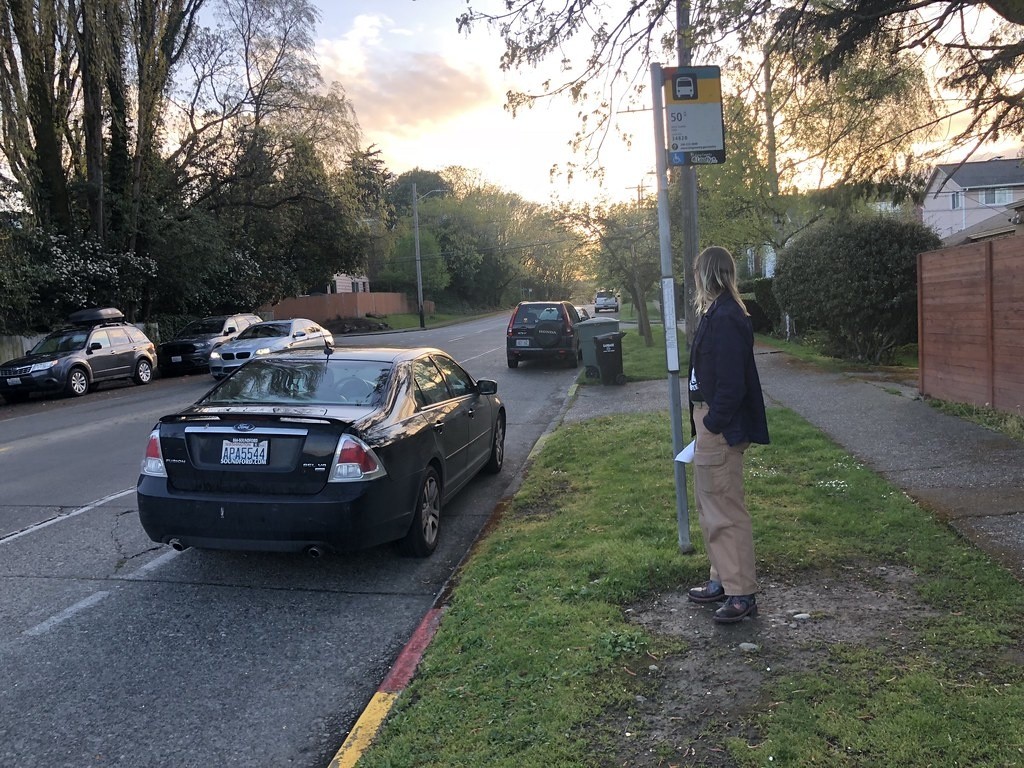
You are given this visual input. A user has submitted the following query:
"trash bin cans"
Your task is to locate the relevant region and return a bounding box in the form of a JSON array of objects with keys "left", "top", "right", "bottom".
[
  {"left": 592, "top": 332, "right": 628, "bottom": 386},
  {"left": 574, "top": 317, "right": 621, "bottom": 380}
]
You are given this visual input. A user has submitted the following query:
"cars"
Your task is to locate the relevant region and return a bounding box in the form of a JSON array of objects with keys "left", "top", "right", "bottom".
[
  {"left": 538, "top": 307, "right": 590, "bottom": 326},
  {"left": 154, "top": 311, "right": 261, "bottom": 373},
  {"left": 208, "top": 318, "right": 335, "bottom": 382},
  {"left": 135, "top": 339, "right": 506, "bottom": 564}
]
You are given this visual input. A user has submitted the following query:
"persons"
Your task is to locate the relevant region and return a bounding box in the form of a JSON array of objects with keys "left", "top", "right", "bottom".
[{"left": 683, "top": 243, "right": 772, "bottom": 624}]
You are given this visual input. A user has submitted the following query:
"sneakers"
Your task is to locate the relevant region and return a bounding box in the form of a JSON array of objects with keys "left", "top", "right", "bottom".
[
  {"left": 687, "top": 583, "right": 727, "bottom": 602},
  {"left": 714, "top": 596, "right": 758, "bottom": 623}
]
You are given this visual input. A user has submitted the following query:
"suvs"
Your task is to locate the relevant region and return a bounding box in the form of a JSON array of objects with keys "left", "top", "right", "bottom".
[
  {"left": 593, "top": 289, "right": 621, "bottom": 312},
  {"left": 0, "top": 306, "right": 158, "bottom": 402},
  {"left": 505, "top": 300, "right": 581, "bottom": 369}
]
